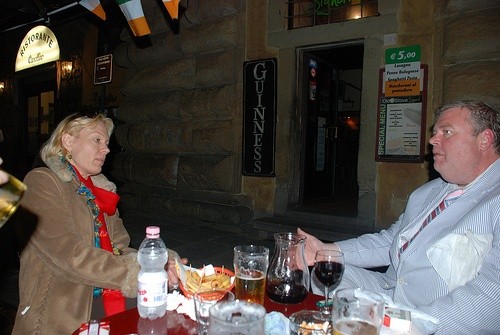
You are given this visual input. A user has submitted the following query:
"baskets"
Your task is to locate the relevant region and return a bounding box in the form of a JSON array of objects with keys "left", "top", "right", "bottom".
[{"left": 180, "top": 266, "right": 236, "bottom": 301}]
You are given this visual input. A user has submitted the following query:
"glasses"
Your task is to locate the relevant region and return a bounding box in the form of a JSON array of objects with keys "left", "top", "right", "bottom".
[{"left": 67, "top": 109, "right": 98, "bottom": 124}]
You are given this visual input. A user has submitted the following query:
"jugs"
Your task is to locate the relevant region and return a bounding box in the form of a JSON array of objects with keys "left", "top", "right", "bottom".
[{"left": 266, "top": 231, "right": 310, "bottom": 304}]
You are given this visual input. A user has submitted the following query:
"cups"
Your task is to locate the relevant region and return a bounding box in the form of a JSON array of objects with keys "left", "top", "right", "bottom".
[
  {"left": 332, "top": 287, "right": 386, "bottom": 335},
  {"left": 289, "top": 310, "right": 332, "bottom": 335},
  {"left": 194, "top": 289, "right": 234, "bottom": 335},
  {"left": 209, "top": 299, "right": 266, "bottom": 335},
  {"left": 232, "top": 244, "right": 269, "bottom": 307},
  {"left": 0, "top": 170, "right": 27, "bottom": 228}
]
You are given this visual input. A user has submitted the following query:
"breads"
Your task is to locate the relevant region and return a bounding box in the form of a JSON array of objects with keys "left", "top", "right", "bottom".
[{"left": 186, "top": 270, "right": 230, "bottom": 298}]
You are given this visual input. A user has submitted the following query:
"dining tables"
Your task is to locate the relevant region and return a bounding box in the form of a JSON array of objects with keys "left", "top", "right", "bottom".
[{"left": 73, "top": 283, "right": 331, "bottom": 335}]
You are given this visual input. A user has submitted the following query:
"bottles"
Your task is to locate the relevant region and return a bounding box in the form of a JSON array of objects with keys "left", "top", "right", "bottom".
[{"left": 136, "top": 226, "right": 168, "bottom": 320}]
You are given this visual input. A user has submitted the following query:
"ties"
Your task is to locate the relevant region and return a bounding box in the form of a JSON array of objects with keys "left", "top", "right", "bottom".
[{"left": 399, "top": 188, "right": 461, "bottom": 254}]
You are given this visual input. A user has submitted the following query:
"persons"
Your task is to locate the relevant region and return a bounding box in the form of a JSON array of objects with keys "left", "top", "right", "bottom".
[
  {"left": 8, "top": 111, "right": 188, "bottom": 335},
  {"left": 284, "top": 100, "right": 500, "bottom": 335}
]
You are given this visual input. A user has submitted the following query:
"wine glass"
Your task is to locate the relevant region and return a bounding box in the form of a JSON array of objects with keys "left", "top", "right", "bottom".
[{"left": 314, "top": 249, "right": 345, "bottom": 321}]
